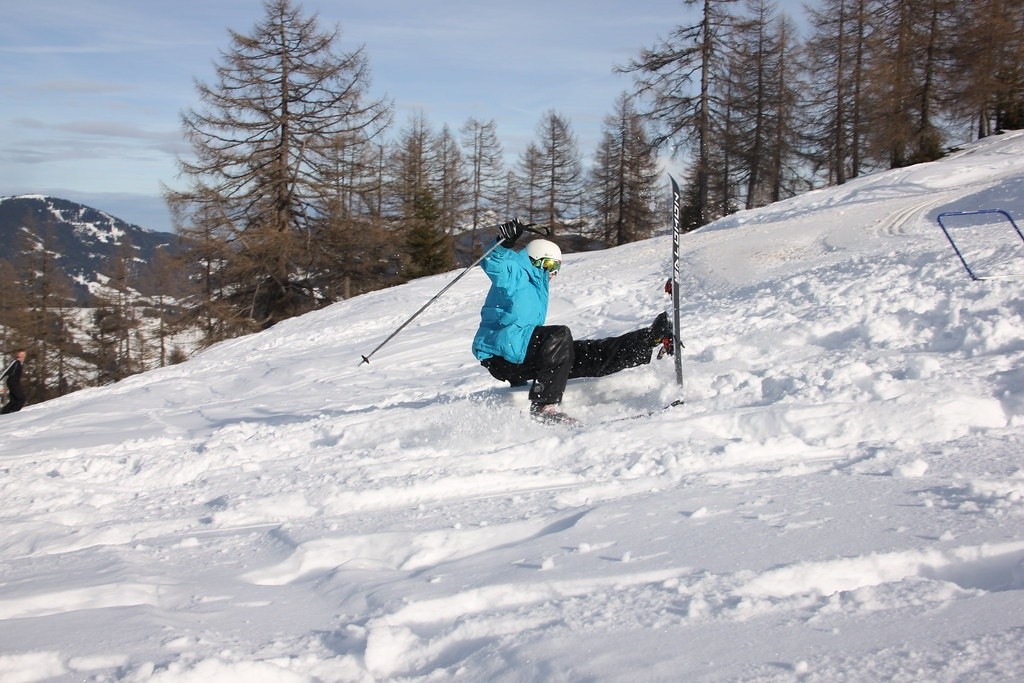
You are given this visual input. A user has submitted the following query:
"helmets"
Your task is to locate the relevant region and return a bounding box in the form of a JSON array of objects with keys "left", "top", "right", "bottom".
[{"left": 525, "top": 239, "right": 562, "bottom": 267}]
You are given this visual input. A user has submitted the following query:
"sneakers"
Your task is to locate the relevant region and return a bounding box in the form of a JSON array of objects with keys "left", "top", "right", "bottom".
[
  {"left": 651, "top": 311, "right": 674, "bottom": 359},
  {"left": 530, "top": 401, "right": 583, "bottom": 430}
]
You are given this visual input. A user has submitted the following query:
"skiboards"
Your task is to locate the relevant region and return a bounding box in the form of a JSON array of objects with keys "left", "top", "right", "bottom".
[
  {"left": 489, "top": 398, "right": 686, "bottom": 456},
  {"left": 666, "top": 171, "right": 685, "bottom": 385}
]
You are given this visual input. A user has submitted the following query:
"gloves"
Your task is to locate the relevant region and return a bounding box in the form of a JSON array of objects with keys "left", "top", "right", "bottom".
[{"left": 496, "top": 218, "right": 523, "bottom": 248}]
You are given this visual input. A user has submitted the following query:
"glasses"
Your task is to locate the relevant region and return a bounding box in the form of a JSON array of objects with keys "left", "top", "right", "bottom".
[{"left": 535, "top": 258, "right": 561, "bottom": 273}]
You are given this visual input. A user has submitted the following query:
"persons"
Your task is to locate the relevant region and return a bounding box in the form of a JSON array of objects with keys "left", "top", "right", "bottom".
[
  {"left": 0, "top": 351, "right": 26, "bottom": 415},
  {"left": 470, "top": 217, "right": 673, "bottom": 427}
]
